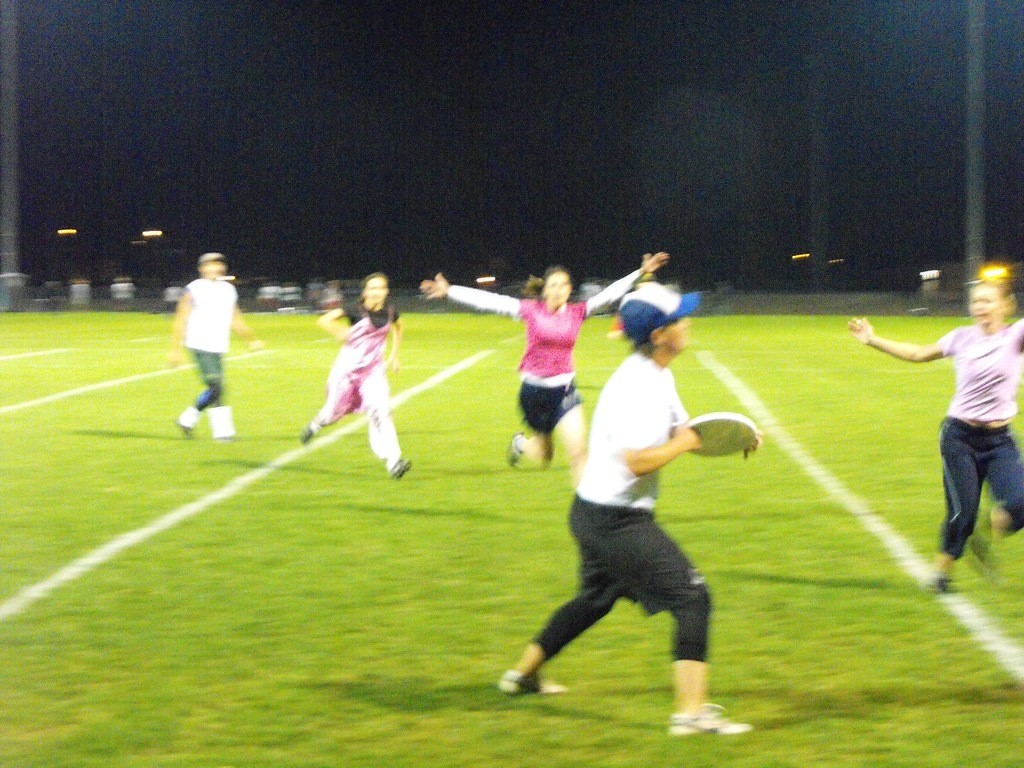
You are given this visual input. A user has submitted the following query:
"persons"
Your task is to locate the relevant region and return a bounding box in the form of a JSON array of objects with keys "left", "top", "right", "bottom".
[
  {"left": 419, "top": 250, "right": 673, "bottom": 468},
  {"left": 298, "top": 271, "right": 412, "bottom": 480},
  {"left": 495, "top": 281, "right": 768, "bottom": 736},
  {"left": 168, "top": 252, "right": 254, "bottom": 442},
  {"left": 847, "top": 263, "right": 1024, "bottom": 593}
]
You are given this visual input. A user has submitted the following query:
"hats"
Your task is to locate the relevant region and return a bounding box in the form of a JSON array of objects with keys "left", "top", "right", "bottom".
[
  {"left": 196, "top": 253, "right": 226, "bottom": 264},
  {"left": 618, "top": 281, "right": 703, "bottom": 344}
]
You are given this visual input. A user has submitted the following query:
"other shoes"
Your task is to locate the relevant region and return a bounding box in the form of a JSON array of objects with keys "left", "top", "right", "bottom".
[
  {"left": 506, "top": 431, "right": 524, "bottom": 467},
  {"left": 301, "top": 425, "right": 313, "bottom": 445},
  {"left": 390, "top": 459, "right": 412, "bottom": 479},
  {"left": 174, "top": 419, "right": 193, "bottom": 439},
  {"left": 923, "top": 572, "right": 950, "bottom": 592},
  {"left": 971, "top": 536, "right": 996, "bottom": 570}
]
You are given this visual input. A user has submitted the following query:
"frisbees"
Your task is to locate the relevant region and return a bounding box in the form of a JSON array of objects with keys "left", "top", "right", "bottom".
[{"left": 684, "top": 411, "right": 761, "bottom": 458}]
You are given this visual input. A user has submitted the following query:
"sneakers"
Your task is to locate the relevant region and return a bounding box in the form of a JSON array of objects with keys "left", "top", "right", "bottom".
[
  {"left": 499, "top": 670, "right": 569, "bottom": 698},
  {"left": 670, "top": 703, "right": 754, "bottom": 738}
]
